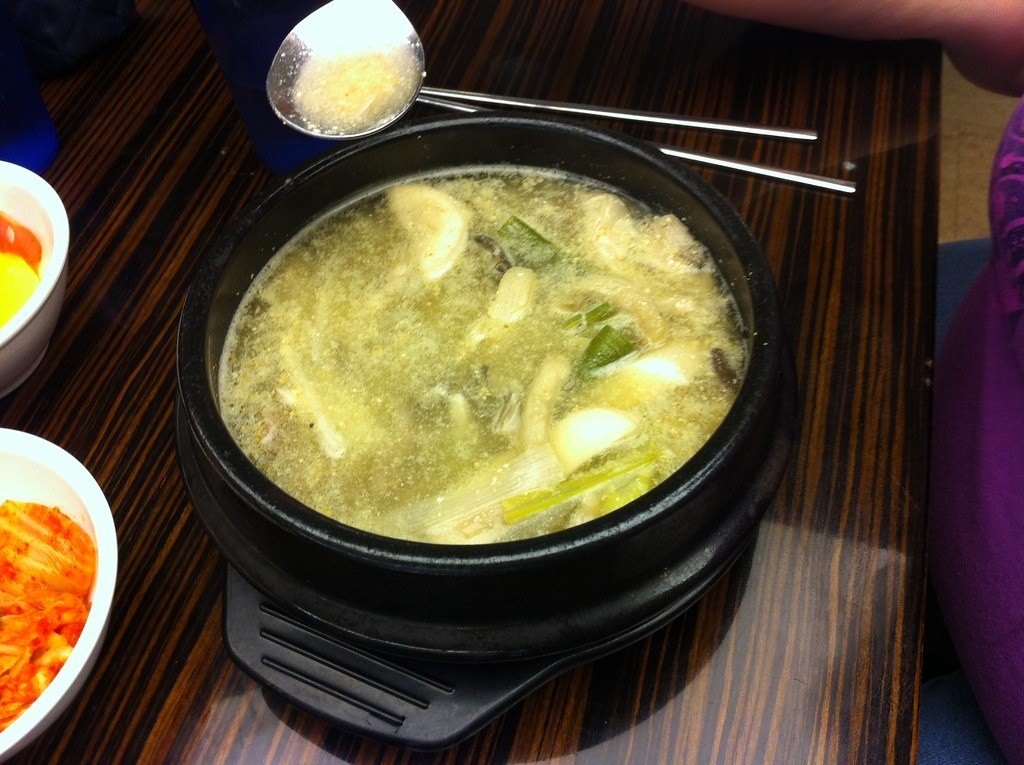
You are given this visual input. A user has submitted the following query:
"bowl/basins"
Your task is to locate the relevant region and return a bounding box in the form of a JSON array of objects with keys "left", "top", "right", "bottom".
[
  {"left": 0, "top": 420, "right": 123, "bottom": 763},
  {"left": 176, "top": 112, "right": 770, "bottom": 589},
  {"left": 0, "top": 154, "right": 71, "bottom": 401}
]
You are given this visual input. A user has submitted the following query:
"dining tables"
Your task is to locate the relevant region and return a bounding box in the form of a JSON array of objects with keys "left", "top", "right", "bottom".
[{"left": 0, "top": 0, "right": 944, "bottom": 765}]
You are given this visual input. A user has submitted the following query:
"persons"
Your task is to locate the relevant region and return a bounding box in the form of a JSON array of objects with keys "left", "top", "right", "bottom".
[{"left": 692, "top": 0, "right": 1024, "bottom": 764}]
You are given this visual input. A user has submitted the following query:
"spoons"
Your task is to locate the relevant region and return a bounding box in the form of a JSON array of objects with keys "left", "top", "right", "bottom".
[{"left": 268, "top": 0, "right": 861, "bottom": 196}]
